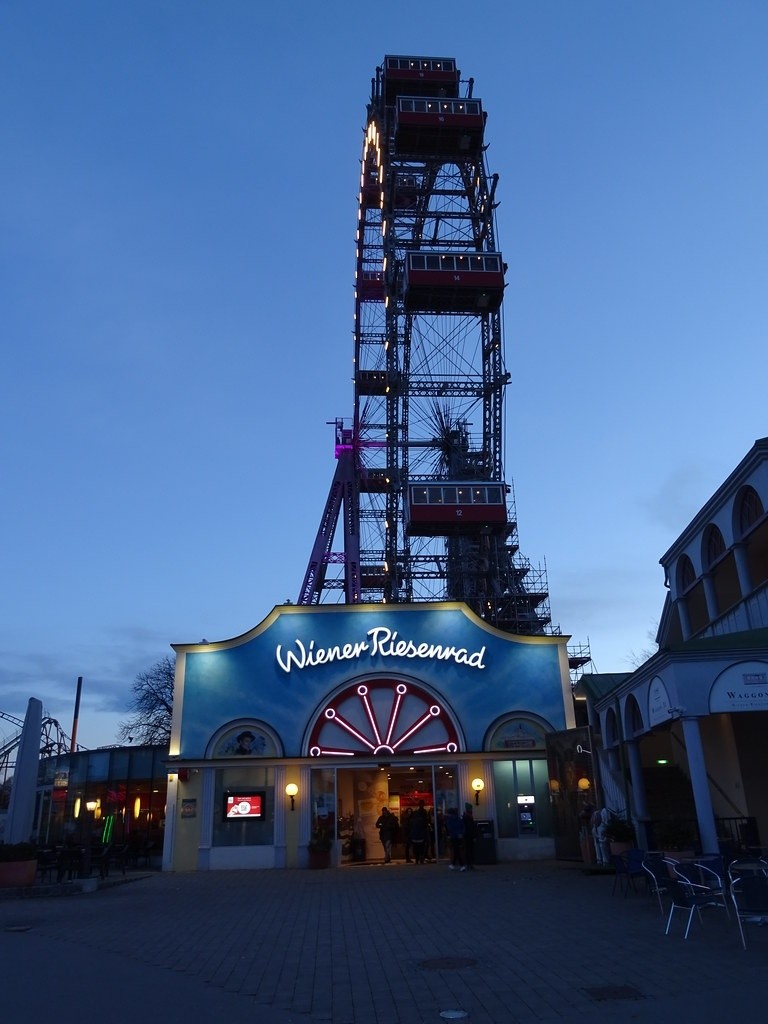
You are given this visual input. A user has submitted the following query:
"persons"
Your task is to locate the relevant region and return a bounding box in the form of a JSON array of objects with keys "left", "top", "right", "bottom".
[
  {"left": 375, "top": 797, "right": 480, "bottom": 872},
  {"left": 236, "top": 730, "right": 257, "bottom": 755}
]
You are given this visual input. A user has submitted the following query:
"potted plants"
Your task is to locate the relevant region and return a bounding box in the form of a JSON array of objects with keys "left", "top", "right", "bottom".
[
  {"left": 0, "top": 840, "right": 46, "bottom": 887},
  {"left": 603, "top": 807, "right": 631, "bottom": 853}
]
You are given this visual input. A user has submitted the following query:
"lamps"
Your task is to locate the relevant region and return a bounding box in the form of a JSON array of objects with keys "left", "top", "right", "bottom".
[
  {"left": 285, "top": 783, "right": 298, "bottom": 811},
  {"left": 577, "top": 776, "right": 592, "bottom": 800},
  {"left": 471, "top": 777, "right": 484, "bottom": 806}
]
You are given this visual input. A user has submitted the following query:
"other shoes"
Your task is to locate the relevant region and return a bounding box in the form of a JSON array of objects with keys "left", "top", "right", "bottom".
[
  {"left": 460, "top": 867, "right": 465, "bottom": 872},
  {"left": 449, "top": 864, "right": 455, "bottom": 870}
]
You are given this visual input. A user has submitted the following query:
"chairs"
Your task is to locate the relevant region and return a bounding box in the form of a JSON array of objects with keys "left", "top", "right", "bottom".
[
  {"left": 641, "top": 856, "right": 702, "bottom": 920},
  {"left": 608, "top": 853, "right": 637, "bottom": 897},
  {"left": 727, "top": 859, "right": 768, "bottom": 951},
  {"left": 666, "top": 859, "right": 739, "bottom": 940},
  {"left": 621, "top": 848, "right": 651, "bottom": 898},
  {"left": 26, "top": 834, "right": 128, "bottom": 880}
]
